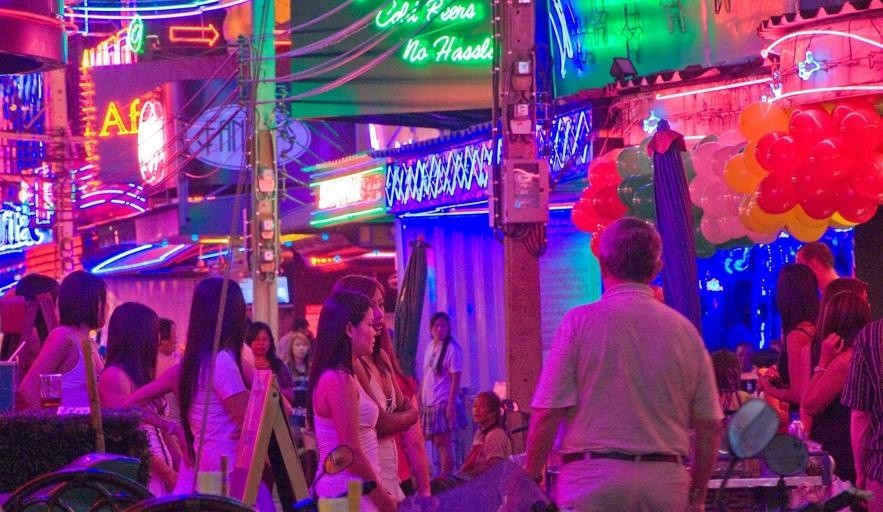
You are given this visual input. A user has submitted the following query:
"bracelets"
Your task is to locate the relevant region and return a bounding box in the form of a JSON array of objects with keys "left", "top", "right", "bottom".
[{"left": 533, "top": 476, "right": 542, "bottom": 485}]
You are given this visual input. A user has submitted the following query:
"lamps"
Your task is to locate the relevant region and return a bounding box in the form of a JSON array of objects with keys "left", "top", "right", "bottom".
[
  {"left": 211, "top": 243, "right": 226, "bottom": 269},
  {"left": 192, "top": 244, "right": 210, "bottom": 273},
  {"left": 610, "top": 57, "right": 638, "bottom": 82}
]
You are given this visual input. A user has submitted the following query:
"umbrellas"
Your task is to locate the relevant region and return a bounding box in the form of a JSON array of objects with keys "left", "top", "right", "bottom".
[{"left": 644, "top": 119, "right": 704, "bottom": 337}]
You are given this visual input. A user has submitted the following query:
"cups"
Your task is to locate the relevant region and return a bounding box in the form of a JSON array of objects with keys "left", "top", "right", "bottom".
[
  {"left": 38, "top": 372, "right": 63, "bottom": 411},
  {"left": 0, "top": 360, "right": 17, "bottom": 410}
]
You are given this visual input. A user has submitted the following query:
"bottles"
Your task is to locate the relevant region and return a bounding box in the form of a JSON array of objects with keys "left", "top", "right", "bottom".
[{"left": 788, "top": 421, "right": 808, "bottom": 441}]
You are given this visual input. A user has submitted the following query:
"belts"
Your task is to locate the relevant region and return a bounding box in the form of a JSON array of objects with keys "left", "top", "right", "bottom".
[{"left": 560, "top": 450, "right": 683, "bottom": 465}]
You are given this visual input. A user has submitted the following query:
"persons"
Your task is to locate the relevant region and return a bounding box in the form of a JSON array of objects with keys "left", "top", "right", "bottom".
[
  {"left": 1, "top": 272, "right": 433, "bottom": 510},
  {"left": 417, "top": 310, "right": 464, "bottom": 473},
  {"left": 710, "top": 241, "right": 883, "bottom": 512},
  {"left": 506, "top": 215, "right": 724, "bottom": 512},
  {"left": 433, "top": 389, "right": 510, "bottom": 490}
]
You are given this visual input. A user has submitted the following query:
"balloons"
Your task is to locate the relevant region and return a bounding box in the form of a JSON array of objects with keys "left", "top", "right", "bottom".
[{"left": 571, "top": 99, "right": 883, "bottom": 255}]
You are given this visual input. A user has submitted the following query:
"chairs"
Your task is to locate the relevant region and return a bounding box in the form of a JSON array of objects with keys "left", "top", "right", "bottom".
[
  {"left": 121, "top": 493, "right": 262, "bottom": 512},
  {"left": 0, "top": 468, "right": 156, "bottom": 511},
  {"left": 711, "top": 409, "right": 750, "bottom": 479}
]
